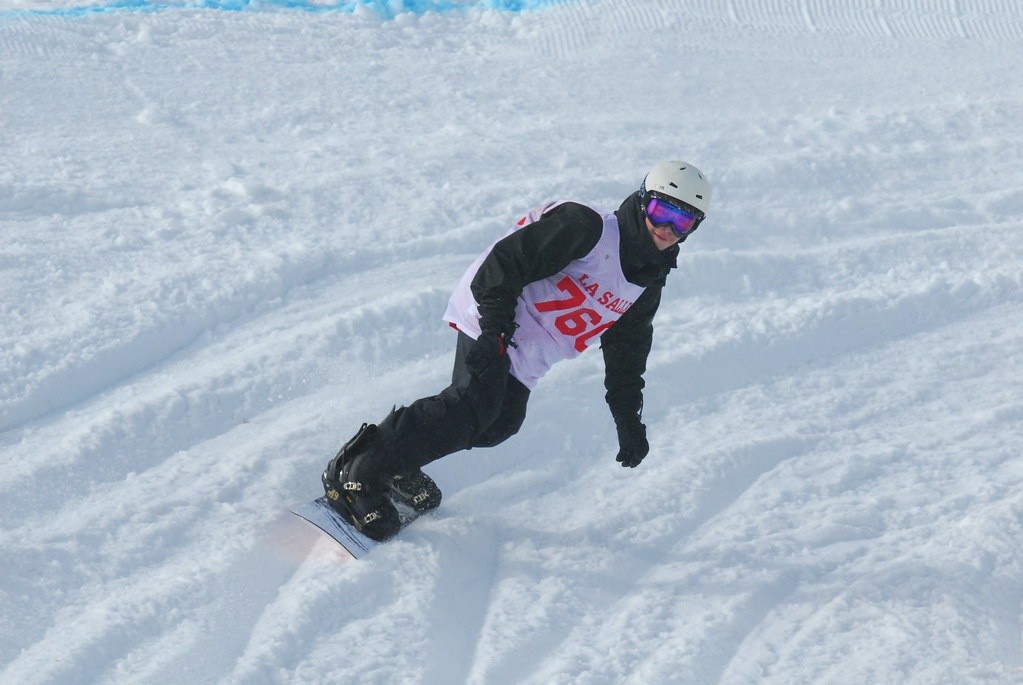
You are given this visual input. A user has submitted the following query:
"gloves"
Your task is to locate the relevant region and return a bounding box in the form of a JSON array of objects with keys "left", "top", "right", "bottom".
[
  {"left": 613, "top": 407, "right": 650, "bottom": 468},
  {"left": 464, "top": 316, "right": 520, "bottom": 367}
]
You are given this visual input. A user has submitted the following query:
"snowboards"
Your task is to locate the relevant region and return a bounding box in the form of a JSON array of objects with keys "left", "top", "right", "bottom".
[{"left": 287, "top": 460, "right": 457, "bottom": 563}]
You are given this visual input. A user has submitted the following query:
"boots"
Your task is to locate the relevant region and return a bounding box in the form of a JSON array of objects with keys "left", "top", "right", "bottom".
[
  {"left": 321, "top": 404, "right": 409, "bottom": 540},
  {"left": 386, "top": 466, "right": 442, "bottom": 514}
]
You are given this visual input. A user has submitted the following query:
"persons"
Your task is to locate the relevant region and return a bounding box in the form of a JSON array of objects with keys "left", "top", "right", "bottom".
[{"left": 322, "top": 159, "right": 712, "bottom": 542}]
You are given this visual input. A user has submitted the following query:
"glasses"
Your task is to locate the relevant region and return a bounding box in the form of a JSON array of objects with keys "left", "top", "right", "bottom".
[{"left": 644, "top": 196, "right": 697, "bottom": 239}]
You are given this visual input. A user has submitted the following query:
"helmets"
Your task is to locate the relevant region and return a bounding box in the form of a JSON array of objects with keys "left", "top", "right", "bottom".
[{"left": 641, "top": 160, "right": 711, "bottom": 230}]
